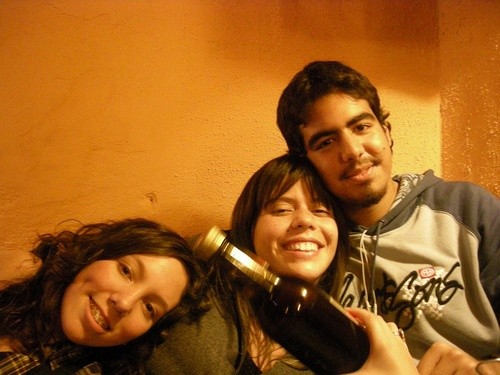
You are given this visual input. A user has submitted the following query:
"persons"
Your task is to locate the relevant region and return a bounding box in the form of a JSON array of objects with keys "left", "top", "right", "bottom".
[
  {"left": 276, "top": 60, "right": 500, "bottom": 375},
  {"left": 148, "top": 153, "right": 418, "bottom": 375},
  {"left": 1, "top": 218, "right": 213, "bottom": 375}
]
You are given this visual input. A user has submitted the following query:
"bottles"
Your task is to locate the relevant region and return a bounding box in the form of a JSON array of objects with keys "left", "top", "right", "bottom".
[{"left": 192, "top": 226, "right": 372, "bottom": 375}]
